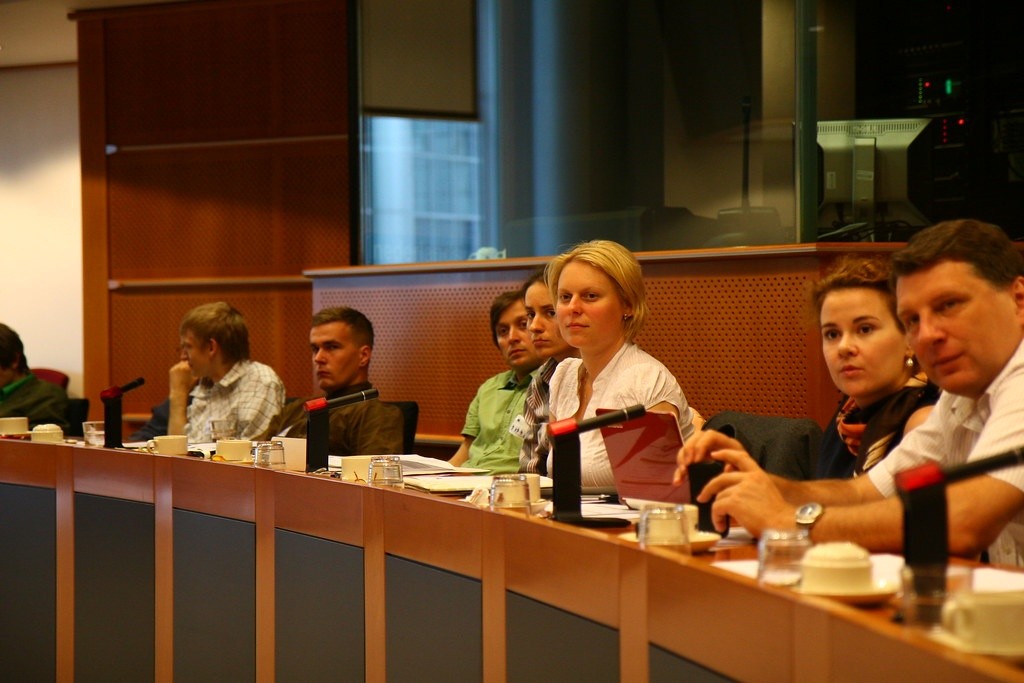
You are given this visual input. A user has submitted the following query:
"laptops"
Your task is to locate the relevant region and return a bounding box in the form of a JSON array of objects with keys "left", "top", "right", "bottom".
[{"left": 596, "top": 409, "right": 692, "bottom": 507}]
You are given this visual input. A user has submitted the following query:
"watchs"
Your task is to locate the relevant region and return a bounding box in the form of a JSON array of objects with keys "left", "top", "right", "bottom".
[{"left": 795, "top": 502, "right": 825, "bottom": 539}]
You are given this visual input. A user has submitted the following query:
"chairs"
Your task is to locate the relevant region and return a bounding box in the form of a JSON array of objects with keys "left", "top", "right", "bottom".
[
  {"left": 63, "top": 397, "right": 91, "bottom": 439},
  {"left": 383, "top": 401, "right": 419, "bottom": 454},
  {"left": 698, "top": 411, "right": 826, "bottom": 482}
]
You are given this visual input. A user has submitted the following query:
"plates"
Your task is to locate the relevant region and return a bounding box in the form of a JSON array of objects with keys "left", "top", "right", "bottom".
[
  {"left": 3, "top": 431, "right": 34, "bottom": 436},
  {"left": 931, "top": 627, "right": 1024, "bottom": 663},
  {"left": 618, "top": 532, "right": 720, "bottom": 550},
  {"left": 792, "top": 581, "right": 900, "bottom": 604},
  {"left": 529, "top": 500, "right": 553, "bottom": 513}
]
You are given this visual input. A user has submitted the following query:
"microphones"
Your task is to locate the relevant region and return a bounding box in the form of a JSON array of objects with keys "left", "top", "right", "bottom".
[
  {"left": 941, "top": 445, "right": 1024, "bottom": 482},
  {"left": 575, "top": 404, "right": 646, "bottom": 432},
  {"left": 120, "top": 377, "right": 144, "bottom": 393},
  {"left": 328, "top": 388, "right": 379, "bottom": 410}
]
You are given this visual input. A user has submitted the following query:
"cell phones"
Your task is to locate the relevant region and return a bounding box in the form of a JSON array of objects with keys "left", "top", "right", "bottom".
[{"left": 688, "top": 461, "right": 729, "bottom": 538}]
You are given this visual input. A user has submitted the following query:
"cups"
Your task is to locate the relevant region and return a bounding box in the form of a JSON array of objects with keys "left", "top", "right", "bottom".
[
  {"left": 146, "top": 435, "right": 188, "bottom": 455},
  {"left": 799, "top": 539, "right": 874, "bottom": 591},
  {"left": 253, "top": 442, "right": 285, "bottom": 470},
  {"left": 81, "top": 421, "right": 106, "bottom": 448},
  {"left": 215, "top": 440, "right": 252, "bottom": 462},
  {"left": 670, "top": 505, "right": 700, "bottom": 534},
  {"left": 942, "top": 590, "right": 1024, "bottom": 656},
  {"left": 523, "top": 474, "right": 541, "bottom": 502},
  {"left": 367, "top": 456, "right": 406, "bottom": 490},
  {"left": 637, "top": 504, "right": 692, "bottom": 558},
  {"left": 0, "top": 417, "right": 29, "bottom": 435},
  {"left": 490, "top": 474, "right": 532, "bottom": 516},
  {"left": 31, "top": 424, "right": 64, "bottom": 444},
  {"left": 756, "top": 529, "right": 813, "bottom": 588}
]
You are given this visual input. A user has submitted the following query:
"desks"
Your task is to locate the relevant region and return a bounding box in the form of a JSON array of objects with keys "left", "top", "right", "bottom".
[{"left": 1, "top": 439, "right": 1024, "bottom": 683}]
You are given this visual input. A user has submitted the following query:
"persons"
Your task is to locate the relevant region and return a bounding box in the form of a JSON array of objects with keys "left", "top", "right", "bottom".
[
  {"left": 180, "top": 302, "right": 286, "bottom": 444},
  {"left": 250, "top": 304, "right": 405, "bottom": 474},
  {"left": 0, "top": 321, "right": 80, "bottom": 433},
  {"left": 126, "top": 338, "right": 202, "bottom": 443},
  {"left": 544, "top": 238, "right": 696, "bottom": 496},
  {"left": 447, "top": 291, "right": 551, "bottom": 475},
  {"left": 810, "top": 254, "right": 941, "bottom": 479},
  {"left": 519, "top": 273, "right": 582, "bottom": 475},
  {"left": 673, "top": 219, "right": 1024, "bottom": 565}
]
